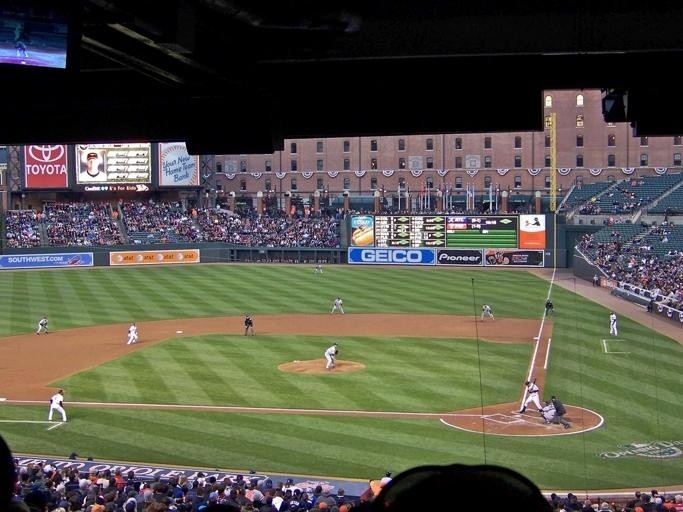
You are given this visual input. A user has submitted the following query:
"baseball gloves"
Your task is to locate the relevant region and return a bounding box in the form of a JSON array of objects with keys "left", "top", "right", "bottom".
[{"left": 334, "top": 350, "right": 337, "bottom": 354}]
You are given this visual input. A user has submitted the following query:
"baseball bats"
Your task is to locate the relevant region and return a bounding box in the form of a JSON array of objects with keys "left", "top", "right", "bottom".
[{"left": 531, "top": 378, "right": 536, "bottom": 390}]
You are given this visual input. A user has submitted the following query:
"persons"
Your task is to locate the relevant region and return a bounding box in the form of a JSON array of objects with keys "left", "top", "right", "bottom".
[
  {"left": 14, "top": 40, "right": 28, "bottom": 58},
  {"left": 79, "top": 152, "right": 107, "bottom": 181}
]
